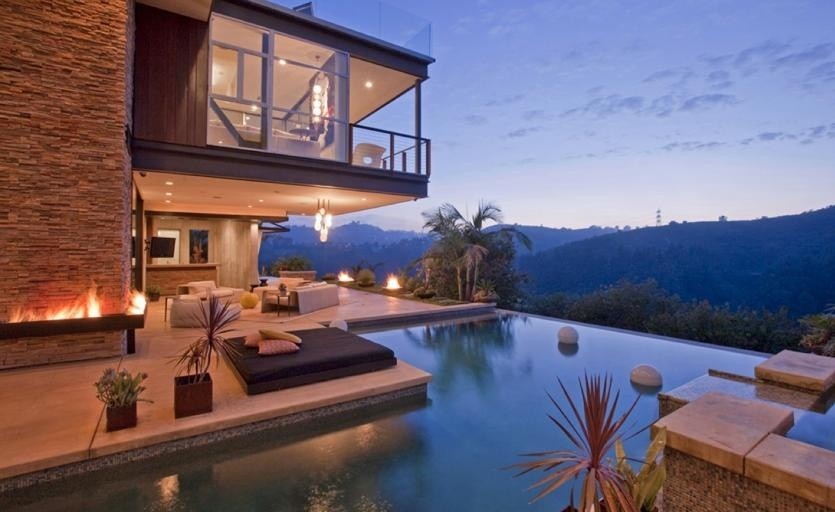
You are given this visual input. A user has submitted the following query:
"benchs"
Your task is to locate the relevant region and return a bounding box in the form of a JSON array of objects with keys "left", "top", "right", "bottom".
[{"left": 163, "top": 278, "right": 341, "bottom": 329}]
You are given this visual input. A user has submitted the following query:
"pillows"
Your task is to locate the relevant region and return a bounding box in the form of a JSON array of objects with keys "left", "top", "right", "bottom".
[{"left": 245, "top": 327, "right": 302, "bottom": 355}]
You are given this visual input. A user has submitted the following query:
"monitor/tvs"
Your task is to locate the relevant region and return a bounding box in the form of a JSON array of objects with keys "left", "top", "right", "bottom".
[{"left": 150, "top": 237, "right": 176, "bottom": 258}]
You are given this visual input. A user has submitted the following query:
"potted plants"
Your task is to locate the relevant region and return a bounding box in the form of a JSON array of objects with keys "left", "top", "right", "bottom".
[{"left": 94, "top": 286, "right": 247, "bottom": 433}]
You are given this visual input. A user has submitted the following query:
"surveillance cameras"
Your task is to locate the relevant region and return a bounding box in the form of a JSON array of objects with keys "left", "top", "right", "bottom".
[{"left": 139, "top": 171, "right": 147, "bottom": 177}]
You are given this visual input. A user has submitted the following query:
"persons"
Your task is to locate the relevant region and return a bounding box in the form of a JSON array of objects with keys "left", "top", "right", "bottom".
[{"left": 192, "top": 239, "right": 204, "bottom": 263}]
